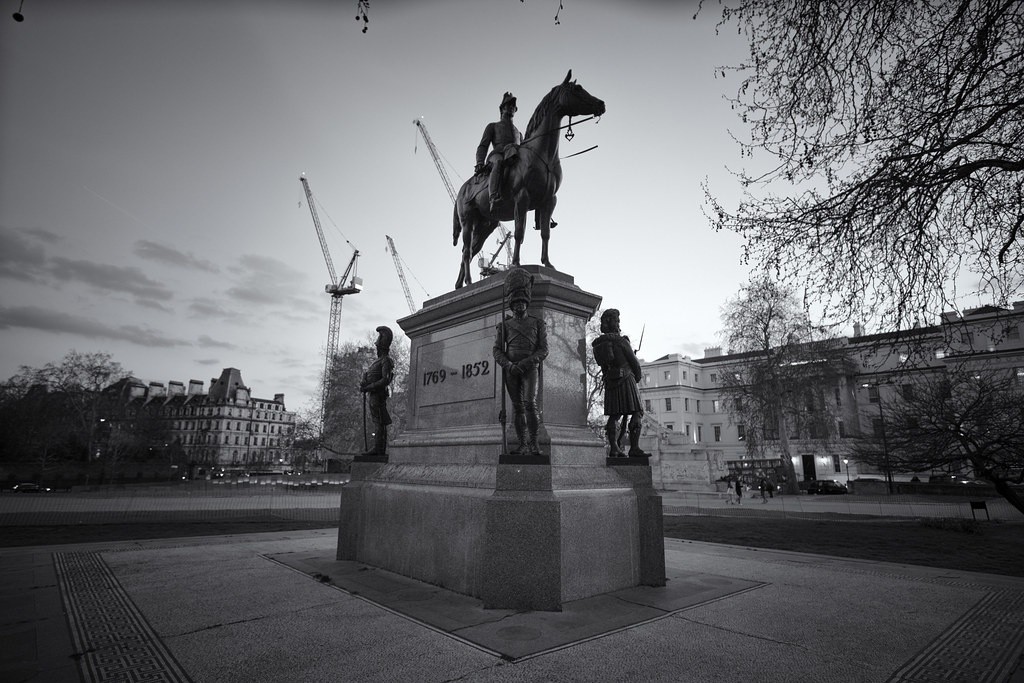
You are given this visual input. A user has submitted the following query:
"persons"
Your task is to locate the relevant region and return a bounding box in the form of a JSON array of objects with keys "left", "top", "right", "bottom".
[
  {"left": 725, "top": 476, "right": 775, "bottom": 506},
  {"left": 359, "top": 327, "right": 394, "bottom": 456},
  {"left": 592, "top": 309, "right": 653, "bottom": 458},
  {"left": 475, "top": 93, "right": 558, "bottom": 230},
  {"left": 491, "top": 268, "right": 549, "bottom": 455}
]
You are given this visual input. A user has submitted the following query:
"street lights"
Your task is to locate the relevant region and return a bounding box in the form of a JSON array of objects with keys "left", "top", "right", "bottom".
[
  {"left": 842, "top": 457, "right": 850, "bottom": 481},
  {"left": 99, "top": 417, "right": 112, "bottom": 485},
  {"left": 244, "top": 401, "right": 257, "bottom": 475},
  {"left": 860, "top": 383, "right": 894, "bottom": 495}
]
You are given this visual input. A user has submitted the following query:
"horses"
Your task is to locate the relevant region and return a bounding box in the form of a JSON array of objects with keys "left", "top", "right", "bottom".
[{"left": 452, "top": 68, "right": 606, "bottom": 290}]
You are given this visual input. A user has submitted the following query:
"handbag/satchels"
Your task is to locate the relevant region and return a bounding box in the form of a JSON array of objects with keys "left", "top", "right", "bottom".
[{"left": 727, "top": 486, "right": 733, "bottom": 495}]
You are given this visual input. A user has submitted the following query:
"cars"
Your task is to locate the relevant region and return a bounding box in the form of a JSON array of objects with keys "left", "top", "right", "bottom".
[
  {"left": 929, "top": 474, "right": 975, "bottom": 486},
  {"left": 12, "top": 483, "right": 52, "bottom": 494},
  {"left": 807, "top": 479, "right": 848, "bottom": 496}
]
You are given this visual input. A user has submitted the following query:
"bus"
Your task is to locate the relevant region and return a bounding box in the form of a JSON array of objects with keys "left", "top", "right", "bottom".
[{"left": 726, "top": 458, "right": 786, "bottom": 492}]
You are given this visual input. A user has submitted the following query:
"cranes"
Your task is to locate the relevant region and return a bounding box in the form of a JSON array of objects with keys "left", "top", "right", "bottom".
[
  {"left": 384, "top": 236, "right": 427, "bottom": 315},
  {"left": 413, "top": 116, "right": 514, "bottom": 281},
  {"left": 297, "top": 174, "right": 361, "bottom": 436}
]
